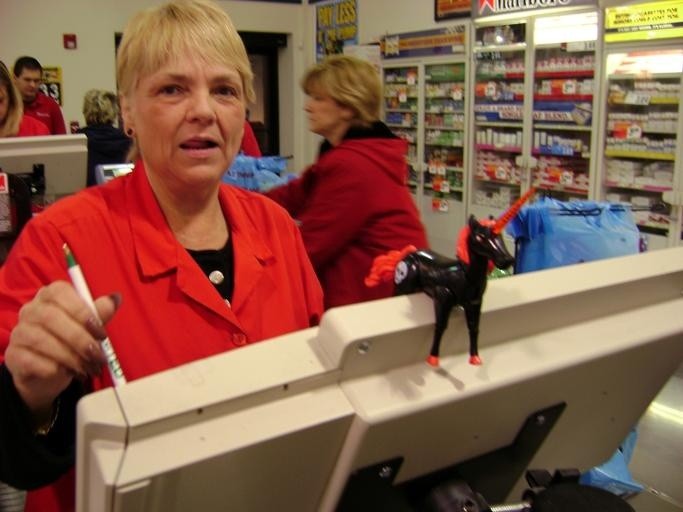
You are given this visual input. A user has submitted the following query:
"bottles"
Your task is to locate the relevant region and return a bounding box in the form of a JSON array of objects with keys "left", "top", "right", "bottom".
[
  {"left": 606, "top": 137, "right": 676, "bottom": 152},
  {"left": 474, "top": 128, "right": 523, "bottom": 149},
  {"left": 531, "top": 130, "right": 584, "bottom": 153},
  {"left": 608, "top": 111, "right": 677, "bottom": 130},
  {"left": 633, "top": 81, "right": 680, "bottom": 97},
  {"left": 28, "top": 162, "right": 46, "bottom": 208}
]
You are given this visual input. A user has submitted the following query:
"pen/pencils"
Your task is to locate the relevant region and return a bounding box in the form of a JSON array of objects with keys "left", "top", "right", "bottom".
[{"left": 62, "top": 242, "right": 128, "bottom": 388}]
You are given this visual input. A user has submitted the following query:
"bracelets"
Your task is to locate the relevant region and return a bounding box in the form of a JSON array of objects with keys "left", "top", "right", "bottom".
[{"left": 35, "top": 401, "right": 60, "bottom": 436}]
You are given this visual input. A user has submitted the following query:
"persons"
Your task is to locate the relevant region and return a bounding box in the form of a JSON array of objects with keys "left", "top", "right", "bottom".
[
  {"left": 13, "top": 56, "right": 66, "bottom": 136},
  {"left": 0, "top": 0, "right": 325, "bottom": 512},
  {"left": 75, "top": 88, "right": 132, "bottom": 188},
  {"left": 260, "top": 54, "right": 429, "bottom": 311},
  {"left": 0, "top": 61, "right": 50, "bottom": 137},
  {"left": 240, "top": 119, "right": 262, "bottom": 158}
]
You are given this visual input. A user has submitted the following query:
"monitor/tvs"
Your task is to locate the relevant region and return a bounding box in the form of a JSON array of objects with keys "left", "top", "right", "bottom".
[
  {"left": 63, "top": 249, "right": 682, "bottom": 512},
  {"left": 0, "top": 134, "right": 94, "bottom": 247}
]
